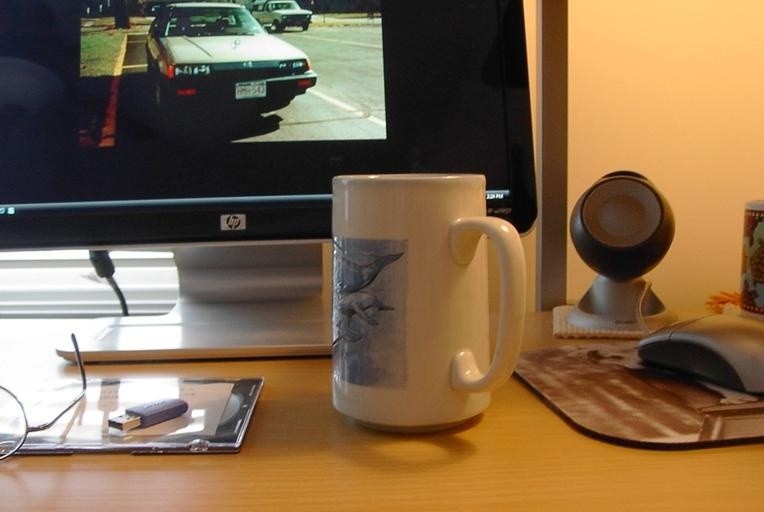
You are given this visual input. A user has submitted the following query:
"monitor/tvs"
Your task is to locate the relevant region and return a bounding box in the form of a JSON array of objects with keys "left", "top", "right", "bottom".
[{"left": 0, "top": 0, "right": 539, "bottom": 363}]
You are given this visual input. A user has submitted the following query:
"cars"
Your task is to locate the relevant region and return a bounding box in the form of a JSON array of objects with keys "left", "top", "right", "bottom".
[{"left": 145, "top": 0, "right": 317, "bottom": 117}]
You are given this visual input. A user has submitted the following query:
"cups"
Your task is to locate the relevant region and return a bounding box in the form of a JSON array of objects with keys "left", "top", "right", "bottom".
[{"left": 333, "top": 175, "right": 526, "bottom": 432}]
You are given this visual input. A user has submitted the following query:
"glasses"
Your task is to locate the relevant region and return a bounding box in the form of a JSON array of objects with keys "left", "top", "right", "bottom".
[{"left": 0, "top": 333, "right": 86, "bottom": 461}]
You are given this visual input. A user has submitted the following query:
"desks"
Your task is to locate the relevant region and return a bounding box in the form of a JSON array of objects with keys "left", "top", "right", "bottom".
[{"left": 0, "top": 297, "right": 761, "bottom": 511}]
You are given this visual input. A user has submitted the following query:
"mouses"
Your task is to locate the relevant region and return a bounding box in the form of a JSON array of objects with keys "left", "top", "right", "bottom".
[{"left": 634, "top": 313, "right": 764, "bottom": 397}]
